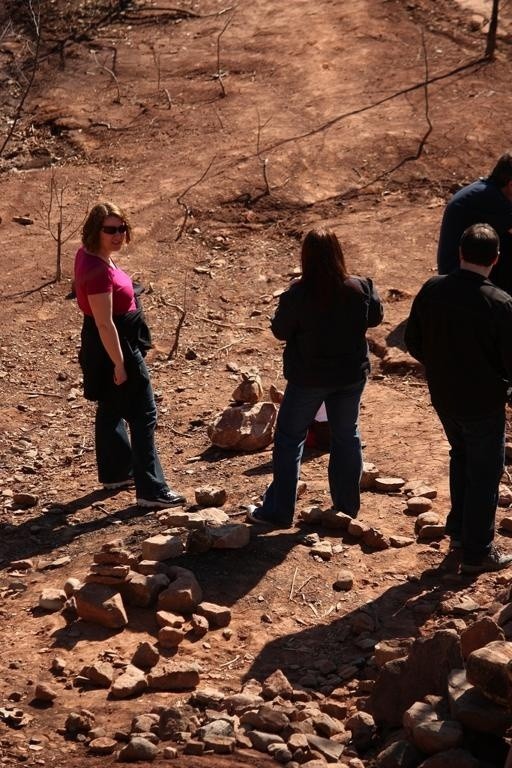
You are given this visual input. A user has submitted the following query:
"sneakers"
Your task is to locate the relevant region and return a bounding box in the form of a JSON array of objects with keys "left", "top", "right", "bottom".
[
  {"left": 100, "top": 469, "right": 138, "bottom": 491},
  {"left": 134, "top": 486, "right": 187, "bottom": 509},
  {"left": 450, "top": 529, "right": 512, "bottom": 574},
  {"left": 246, "top": 506, "right": 293, "bottom": 529}
]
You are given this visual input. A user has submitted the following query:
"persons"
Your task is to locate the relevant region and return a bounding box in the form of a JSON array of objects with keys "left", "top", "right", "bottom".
[
  {"left": 247, "top": 228, "right": 385, "bottom": 531},
  {"left": 70, "top": 202, "right": 187, "bottom": 512},
  {"left": 403, "top": 224, "right": 511, "bottom": 577},
  {"left": 433, "top": 153, "right": 510, "bottom": 295}
]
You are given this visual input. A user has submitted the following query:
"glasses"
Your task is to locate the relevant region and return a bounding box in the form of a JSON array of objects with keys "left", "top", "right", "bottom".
[{"left": 96, "top": 224, "right": 127, "bottom": 235}]
